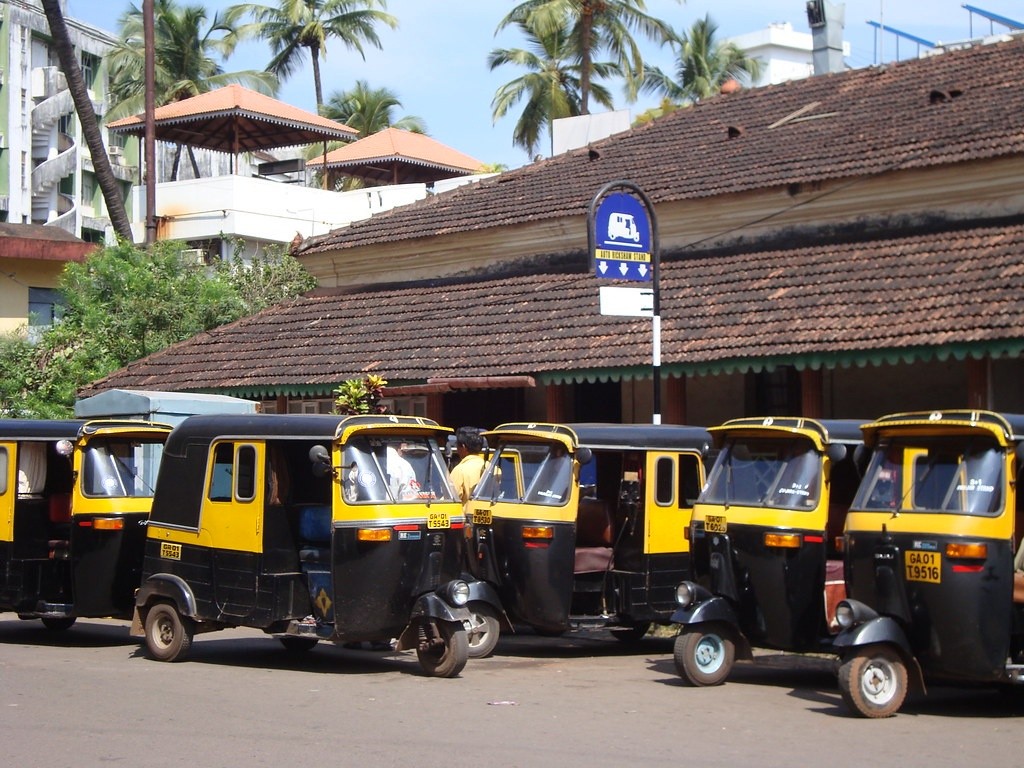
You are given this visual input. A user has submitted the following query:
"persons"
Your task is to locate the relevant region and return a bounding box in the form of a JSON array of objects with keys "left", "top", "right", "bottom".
[
  {"left": 447, "top": 428, "right": 502, "bottom": 554},
  {"left": 386, "top": 443, "right": 417, "bottom": 501}
]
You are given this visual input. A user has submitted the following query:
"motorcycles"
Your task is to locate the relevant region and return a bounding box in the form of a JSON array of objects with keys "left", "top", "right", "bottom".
[
  {"left": 133, "top": 411, "right": 472, "bottom": 680},
  {"left": 0, "top": 418, "right": 175, "bottom": 633},
  {"left": 444, "top": 420, "right": 719, "bottom": 659},
  {"left": 830, "top": 407, "right": 1024, "bottom": 720},
  {"left": 670, "top": 417, "right": 967, "bottom": 690},
  {"left": 401, "top": 447, "right": 525, "bottom": 503}
]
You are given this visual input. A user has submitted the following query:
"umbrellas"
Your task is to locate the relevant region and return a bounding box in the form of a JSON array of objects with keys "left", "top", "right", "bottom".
[
  {"left": 106, "top": 84, "right": 357, "bottom": 177},
  {"left": 304, "top": 127, "right": 487, "bottom": 185}
]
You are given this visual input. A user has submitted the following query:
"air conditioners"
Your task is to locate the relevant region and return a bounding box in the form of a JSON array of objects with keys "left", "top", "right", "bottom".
[
  {"left": 181, "top": 249, "right": 210, "bottom": 266},
  {"left": 108, "top": 146, "right": 123, "bottom": 155}
]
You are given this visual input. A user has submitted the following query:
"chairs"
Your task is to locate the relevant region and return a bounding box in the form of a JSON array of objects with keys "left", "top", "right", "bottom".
[
  {"left": 826, "top": 503, "right": 849, "bottom": 581},
  {"left": 1013, "top": 510, "right": 1024, "bottom": 603},
  {"left": 43, "top": 492, "right": 73, "bottom": 548},
  {"left": 298, "top": 505, "right": 331, "bottom": 564},
  {"left": 575, "top": 497, "right": 615, "bottom": 574}
]
